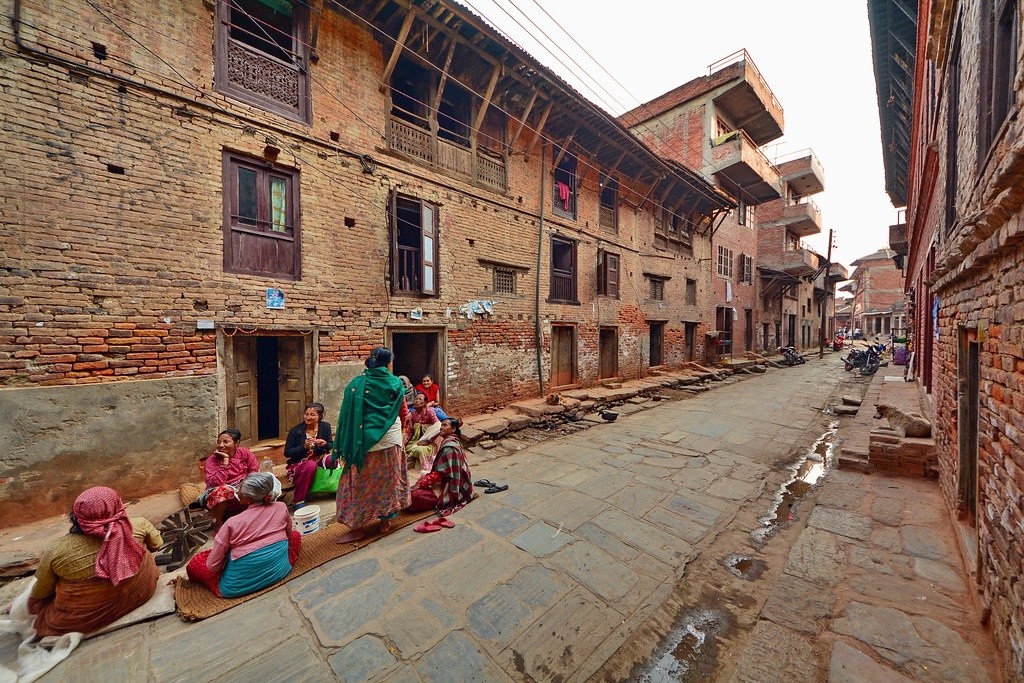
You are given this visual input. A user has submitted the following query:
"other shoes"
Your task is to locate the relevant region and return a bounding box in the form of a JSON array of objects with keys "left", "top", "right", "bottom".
[{"left": 294, "top": 503, "right": 305, "bottom": 511}]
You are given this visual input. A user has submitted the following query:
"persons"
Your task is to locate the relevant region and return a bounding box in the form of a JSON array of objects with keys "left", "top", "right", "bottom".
[
  {"left": 283, "top": 402, "right": 338, "bottom": 510},
  {"left": 401, "top": 417, "right": 478, "bottom": 517},
  {"left": 203, "top": 428, "right": 260, "bottom": 537},
  {"left": 27, "top": 486, "right": 164, "bottom": 638},
  {"left": 414, "top": 374, "right": 449, "bottom": 420},
  {"left": 331, "top": 346, "right": 412, "bottom": 544},
  {"left": 185, "top": 472, "right": 301, "bottom": 599},
  {"left": 398, "top": 392, "right": 444, "bottom": 474},
  {"left": 397, "top": 375, "right": 417, "bottom": 414}
]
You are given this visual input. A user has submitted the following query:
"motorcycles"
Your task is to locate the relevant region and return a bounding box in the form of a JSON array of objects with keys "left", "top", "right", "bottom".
[
  {"left": 777, "top": 338, "right": 806, "bottom": 366},
  {"left": 840, "top": 336, "right": 887, "bottom": 376}
]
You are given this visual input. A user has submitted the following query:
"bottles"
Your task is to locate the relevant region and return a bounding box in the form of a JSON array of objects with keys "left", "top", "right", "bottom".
[{"left": 261, "top": 456, "right": 273, "bottom": 473}]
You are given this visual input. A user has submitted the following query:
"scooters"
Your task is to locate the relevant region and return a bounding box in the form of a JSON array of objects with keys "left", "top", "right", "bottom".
[{"left": 833, "top": 327, "right": 862, "bottom": 352}]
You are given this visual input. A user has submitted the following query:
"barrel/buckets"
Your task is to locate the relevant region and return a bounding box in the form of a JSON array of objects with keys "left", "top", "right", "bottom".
[{"left": 293, "top": 504, "right": 321, "bottom": 535}]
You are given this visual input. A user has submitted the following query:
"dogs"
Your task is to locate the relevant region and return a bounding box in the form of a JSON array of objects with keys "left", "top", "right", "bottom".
[{"left": 873, "top": 403, "right": 931, "bottom": 438}]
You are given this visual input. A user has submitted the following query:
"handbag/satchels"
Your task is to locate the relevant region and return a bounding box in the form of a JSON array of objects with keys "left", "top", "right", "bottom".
[
  {"left": 198, "top": 457, "right": 208, "bottom": 482},
  {"left": 310, "top": 454, "right": 344, "bottom": 493}
]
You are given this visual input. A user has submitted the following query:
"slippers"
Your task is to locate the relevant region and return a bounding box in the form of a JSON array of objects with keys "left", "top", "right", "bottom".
[
  {"left": 474, "top": 478, "right": 496, "bottom": 487},
  {"left": 431, "top": 517, "right": 455, "bottom": 528},
  {"left": 484, "top": 484, "right": 508, "bottom": 493},
  {"left": 414, "top": 521, "right": 442, "bottom": 533}
]
